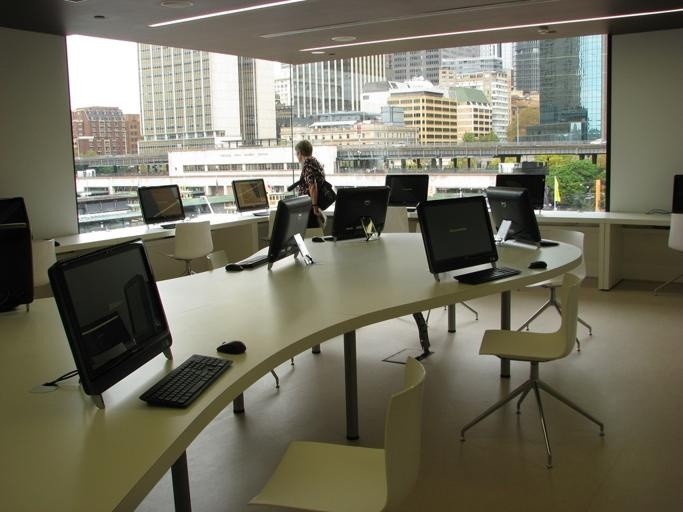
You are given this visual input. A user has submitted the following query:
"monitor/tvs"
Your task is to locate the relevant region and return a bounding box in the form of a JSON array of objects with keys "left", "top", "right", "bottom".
[
  {"left": 268, "top": 195, "right": 313, "bottom": 269},
  {"left": 486, "top": 174, "right": 547, "bottom": 249},
  {"left": 136, "top": 179, "right": 269, "bottom": 229},
  {"left": 0, "top": 196, "right": 33, "bottom": 315},
  {"left": 332, "top": 175, "right": 429, "bottom": 241},
  {"left": 415, "top": 195, "right": 498, "bottom": 281},
  {"left": 47, "top": 238, "right": 173, "bottom": 409}
]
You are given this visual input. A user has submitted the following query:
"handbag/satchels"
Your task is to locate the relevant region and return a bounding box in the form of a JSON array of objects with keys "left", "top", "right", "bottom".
[{"left": 307, "top": 178, "right": 337, "bottom": 211}]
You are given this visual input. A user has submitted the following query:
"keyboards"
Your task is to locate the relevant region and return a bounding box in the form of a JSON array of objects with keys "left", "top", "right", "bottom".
[
  {"left": 516, "top": 239, "right": 559, "bottom": 248},
  {"left": 237, "top": 255, "right": 272, "bottom": 269},
  {"left": 454, "top": 266, "right": 521, "bottom": 286},
  {"left": 139, "top": 354, "right": 233, "bottom": 409},
  {"left": 324, "top": 237, "right": 357, "bottom": 241}
]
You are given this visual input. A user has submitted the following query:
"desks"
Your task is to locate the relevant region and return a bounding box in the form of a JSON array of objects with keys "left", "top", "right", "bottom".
[{"left": 0, "top": 228, "right": 583, "bottom": 512}]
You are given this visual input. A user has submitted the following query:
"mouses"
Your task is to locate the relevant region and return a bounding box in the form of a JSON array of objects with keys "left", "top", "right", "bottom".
[
  {"left": 225, "top": 263, "right": 244, "bottom": 272},
  {"left": 528, "top": 261, "right": 548, "bottom": 269},
  {"left": 216, "top": 340, "right": 246, "bottom": 354},
  {"left": 45, "top": 238, "right": 60, "bottom": 247},
  {"left": 311, "top": 236, "right": 324, "bottom": 242}
]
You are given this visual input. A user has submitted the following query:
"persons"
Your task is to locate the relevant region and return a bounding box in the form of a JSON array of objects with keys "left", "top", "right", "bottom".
[{"left": 295, "top": 141, "right": 325, "bottom": 228}]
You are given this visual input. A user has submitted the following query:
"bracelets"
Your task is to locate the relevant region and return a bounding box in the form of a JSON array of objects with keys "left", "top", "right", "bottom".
[{"left": 312, "top": 205, "right": 317, "bottom": 206}]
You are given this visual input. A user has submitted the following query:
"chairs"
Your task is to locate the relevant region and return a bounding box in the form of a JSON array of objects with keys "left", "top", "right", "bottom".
[
  {"left": 652, "top": 212, "right": 683, "bottom": 297},
  {"left": 459, "top": 272, "right": 604, "bottom": 470},
  {"left": 245, "top": 354, "right": 426, "bottom": 512}
]
